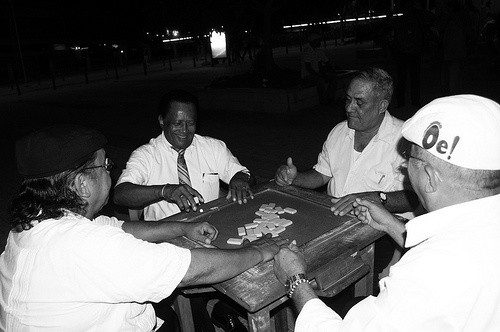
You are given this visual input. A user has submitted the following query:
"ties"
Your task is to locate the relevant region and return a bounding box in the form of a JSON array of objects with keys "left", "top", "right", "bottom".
[{"left": 172, "top": 147, "right": 192, "bottom": 188}]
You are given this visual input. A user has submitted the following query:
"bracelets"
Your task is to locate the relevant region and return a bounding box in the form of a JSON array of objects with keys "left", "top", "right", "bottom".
[
  {"left": 288, "top": 279, "right": 311, "bottom": 298},
  {"left": 162, "top": 184, "right": 170, "bottom": 198}
]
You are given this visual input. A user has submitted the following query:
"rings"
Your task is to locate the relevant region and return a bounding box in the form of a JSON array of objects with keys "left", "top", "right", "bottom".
[{"left": 180, "top": 195, "right": 183, "bottom": 198}]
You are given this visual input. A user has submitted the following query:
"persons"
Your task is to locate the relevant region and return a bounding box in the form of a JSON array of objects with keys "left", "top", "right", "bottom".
[
  {"left": 384, "top": 0, "right": 472, "bottom": 107},
  {"left": 301, "top": 34, "right": 341, "bottom": 106},
  {"left": 0, "top": 93, "right": 290, "bottom": 332},
  {"left": 275, "top": 67, "right": 500, "bottom": 332}
]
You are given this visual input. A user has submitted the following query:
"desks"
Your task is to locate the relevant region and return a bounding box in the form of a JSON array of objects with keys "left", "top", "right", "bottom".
[{"left": 151, "top": 178, "right": 405, "bottom": 332}]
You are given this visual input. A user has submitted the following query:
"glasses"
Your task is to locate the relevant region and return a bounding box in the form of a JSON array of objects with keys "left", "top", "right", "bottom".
[
  {"left": 71, "top": 158, "right": 113, "bottom": 187},
  {"left": 396, "top": 137, "right": 443, "bottom": 182}
]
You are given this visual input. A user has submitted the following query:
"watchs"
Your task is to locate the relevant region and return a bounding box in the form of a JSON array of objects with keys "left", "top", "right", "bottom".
[
  {"left": 285, "top": 274, "right": 306, "bottom": 291},
  {"left": 376, "top": 191, "right": 386, "bottom": 205}
]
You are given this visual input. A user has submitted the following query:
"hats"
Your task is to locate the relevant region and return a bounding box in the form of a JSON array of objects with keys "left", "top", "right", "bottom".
[
  {"left": 400, "top": 94, "right": 500, "bottom": 170},
  {"left": 23, "top": 128, "right": 108, "bottom": 178}
]
